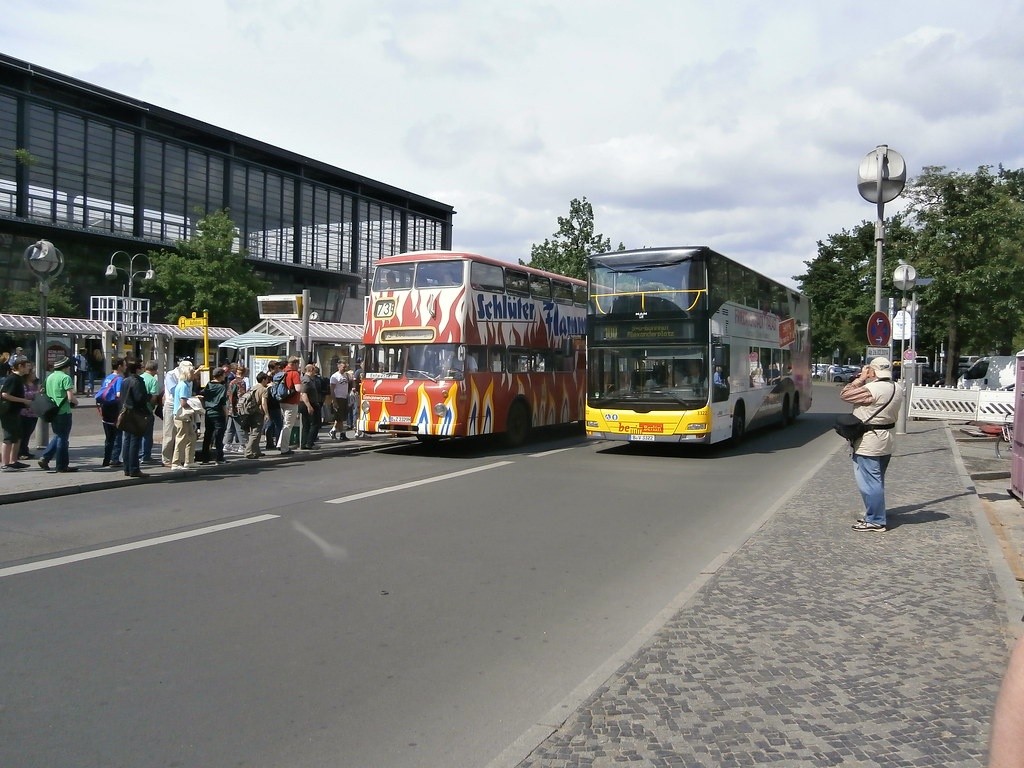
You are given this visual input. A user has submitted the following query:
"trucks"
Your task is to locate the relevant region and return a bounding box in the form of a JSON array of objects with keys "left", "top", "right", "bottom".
[{"left": 957, "top": 356, "right": 1016, "bottom": 391}]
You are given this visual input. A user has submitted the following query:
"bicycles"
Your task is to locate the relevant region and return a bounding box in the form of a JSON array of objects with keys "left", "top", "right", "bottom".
[{"left": 996, "top": 426, "right": 1014, "bottom": 459}]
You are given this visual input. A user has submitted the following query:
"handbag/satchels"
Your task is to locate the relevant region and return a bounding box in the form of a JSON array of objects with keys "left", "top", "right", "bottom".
[
  {"left": 834, "top": 413, "right": 895, "bottom": 441},
  {"left": 154, "top": 403, "right": 164, "bottom": 421},
  {"left": 94, "top": 388, "right": 119, "bottom": 404},
  {"left": 32, "top": 393, "right": 60, "bottom": 423},
  {"left": 117, "top": 408, "right": 150, "bottom": 437}
]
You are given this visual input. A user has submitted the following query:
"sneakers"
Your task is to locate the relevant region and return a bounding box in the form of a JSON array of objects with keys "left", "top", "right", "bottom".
[
  {"left": 857, "top": 515, "right": 866, "bottom": 523},
  {"left": 851, "top": 521, "right": 886, "bottom": 533}
]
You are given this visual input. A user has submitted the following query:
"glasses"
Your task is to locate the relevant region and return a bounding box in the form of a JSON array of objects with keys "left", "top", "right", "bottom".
[{"left": 140, "top": 364, "right": 147, "bottom": 369}]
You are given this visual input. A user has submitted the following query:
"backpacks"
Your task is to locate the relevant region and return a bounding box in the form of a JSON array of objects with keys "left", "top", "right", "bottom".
[
  {"left": 237, "top": 387, "right": 262, "bottom": 415},
  {"left": 271, "top": 368, "right": 297, "bottom": 402}
]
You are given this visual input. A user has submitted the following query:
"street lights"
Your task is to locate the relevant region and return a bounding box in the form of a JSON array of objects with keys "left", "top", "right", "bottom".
[{"left": 105, "top": 251, "right": 155, "bottom": 297}]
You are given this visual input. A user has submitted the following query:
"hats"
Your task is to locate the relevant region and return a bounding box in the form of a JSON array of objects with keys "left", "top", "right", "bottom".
[
  {"left": 16, "top": 347, "right": 23, "bottom": 351},
  {"left": 77, "top": 347, "right": 87, "bottom": 356},
  {"left": 53, "top": 355, "right": 72, "bottom": 370},
  {"left": 211, "top": 368, "right": 225, "bottom": 377},
  {"left": 870, "top": 357, "right": 893, "bottom": 380},
  {"left": 0, "top": 352, "right": 9, "bottom": 364}
]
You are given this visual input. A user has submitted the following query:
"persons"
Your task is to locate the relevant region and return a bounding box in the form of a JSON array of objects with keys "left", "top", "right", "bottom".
[
  {"left": 714, "top": 366, "right": 727, "bottom": 387},
  {"left": 74, "top": 347, "right": 104, "bottom": 397},
  {"left": 645, "top": 361, "right": 664, "bottom": 388},
  {"left": 840, "top": 357, "right": 903, "bottom": 532},
  {"left": 124, "top": 351, "right": 134, "bottom": 361},
  {"left": 328, "top": 360, "right": 384, "bottom": 440},
  {"left": 682, "top": 363, "right": 708, "bottom": 388},
  {"left": 438, "top": 270, "right": 455, "bottom": 285},
  {"left": 198, "top": 356, "right": 321, "bottom": 464},
  {"left": 397, "top": 346, "right": 438, "bottom": 377},
  {"left": 101, "top": 357, "right": 206, "bottom": 470},
  {"left": 380, "top": 272, "right": 402, "bottom": 288},
  {"left": 444, "top": 344, "right": 478, "bottom": 372},
  {"left": 750, "top": 361, "right": 780, "bottom": 388},
  {"left": 408, "top": 271, "right": 428, "bottom": 287},
  {"left": 988, "top": 635, "right": 1024, "bottom": 768},
  {"left": 0, "top": 346, "right": 79, "bottom": 473}
]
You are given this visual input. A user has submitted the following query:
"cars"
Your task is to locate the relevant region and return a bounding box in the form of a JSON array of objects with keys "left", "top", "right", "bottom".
[{"left": 811, "top": 364, "right": 861, "bottom": 382}]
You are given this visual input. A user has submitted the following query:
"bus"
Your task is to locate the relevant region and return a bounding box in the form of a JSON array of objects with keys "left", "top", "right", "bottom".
[
  {"left": 355, "top": 250, "right": 623, "bottom": 448},
  {"left": 585, "top": 246, "right": 813, "bottom": 447}
]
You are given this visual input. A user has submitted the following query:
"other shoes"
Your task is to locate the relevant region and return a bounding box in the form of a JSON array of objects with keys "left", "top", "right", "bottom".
[
  {"left": 10, "top": 462, "right": 30, "bottom": 468},
  {"left": 36, "top": 421, "right": 375, "bottom": 480},
  {"left": 19, "top": 451, "right": 36, "bottom": 460},
  {"left": 0, "top": 465, "right": 20, "bottom": 473}
]
